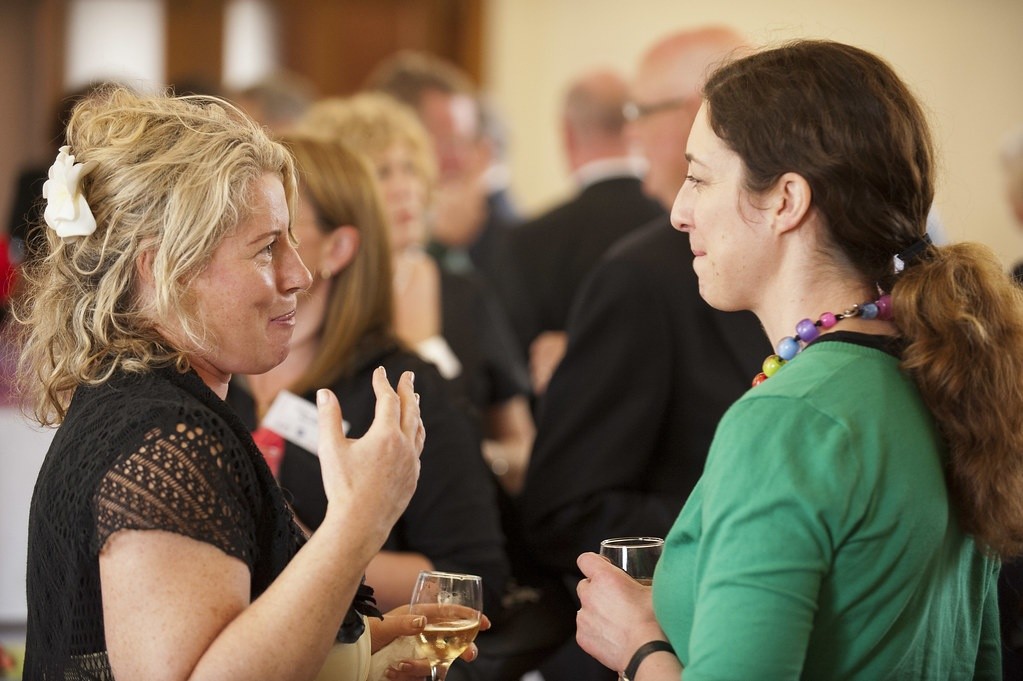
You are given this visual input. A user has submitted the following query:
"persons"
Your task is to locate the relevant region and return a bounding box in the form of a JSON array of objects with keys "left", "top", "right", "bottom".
[
  {"left": 575, "top": 39, "right": 1023, "bottom": 681},
  {"left": 13, "top": 27, "right": 775, "bottom": 681},
  {"left": 20, "top": 90, "right": 490, "bottom": 681}
]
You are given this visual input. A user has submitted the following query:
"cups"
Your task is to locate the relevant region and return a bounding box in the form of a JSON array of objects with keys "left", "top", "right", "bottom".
[{"left": 600, "top": 537, "right": 664, "bottom": 586}]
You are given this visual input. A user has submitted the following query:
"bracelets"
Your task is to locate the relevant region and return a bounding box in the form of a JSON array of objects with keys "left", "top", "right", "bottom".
[{"left": 618, "top": 640, "right": 676, "bottom": 681}]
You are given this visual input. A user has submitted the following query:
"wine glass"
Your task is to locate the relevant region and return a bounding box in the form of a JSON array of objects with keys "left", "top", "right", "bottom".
[{"left": 408, "top": 569, "right": 484, "bottom": 681}]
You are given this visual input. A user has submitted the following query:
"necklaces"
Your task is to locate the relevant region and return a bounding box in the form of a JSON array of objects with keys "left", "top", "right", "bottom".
[{"left": 752, "top": 295, "right": 894, "bottom": 385}]
